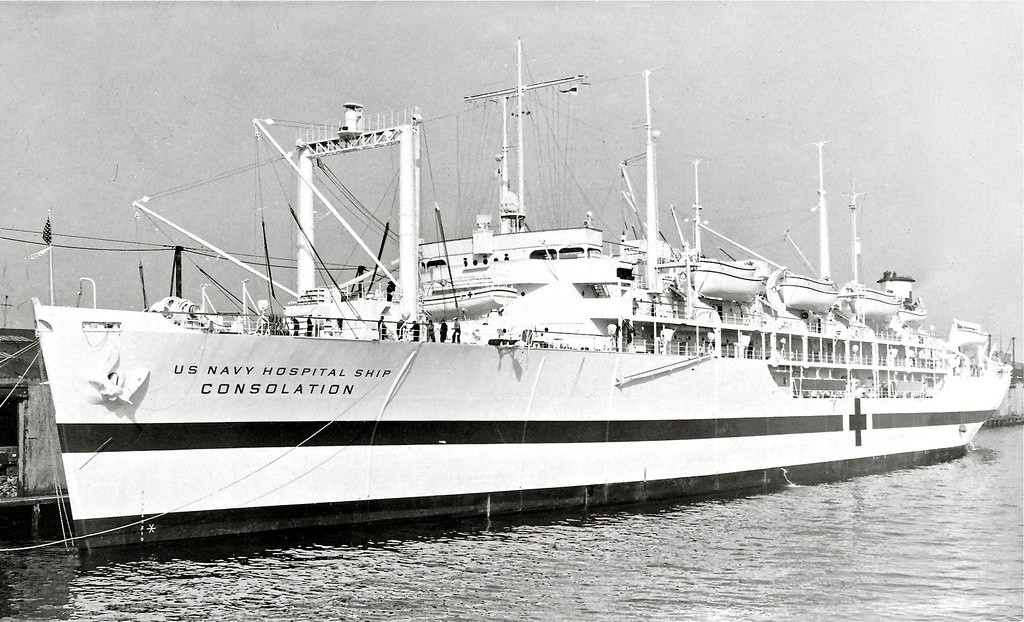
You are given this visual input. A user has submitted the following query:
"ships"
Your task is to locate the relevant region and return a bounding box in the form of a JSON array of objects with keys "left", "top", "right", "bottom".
[{"left": 33, "top": 40, "right": 1014, "bottom": 573}]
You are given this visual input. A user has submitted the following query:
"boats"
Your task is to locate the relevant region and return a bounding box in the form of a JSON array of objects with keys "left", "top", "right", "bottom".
[
  {"left": 778, "top": 274, "right": 836, "bottom": 313},
  {"left": 681, "top": 258, "right": 768, "bottom": 300},
  {"left": 849, "top": 287, "right": 901, "bottom": 319},
  {"left": 953, "top": 329, "right": 989, "bottom": 346}
]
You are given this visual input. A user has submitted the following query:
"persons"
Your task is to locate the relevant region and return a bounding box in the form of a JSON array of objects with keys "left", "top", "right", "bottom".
[{"left": 263, "top": 316, "right": 462, "bottom": 342}]
